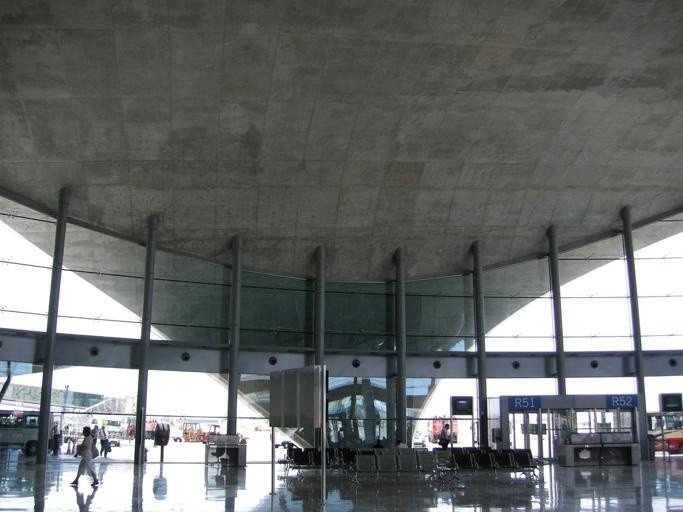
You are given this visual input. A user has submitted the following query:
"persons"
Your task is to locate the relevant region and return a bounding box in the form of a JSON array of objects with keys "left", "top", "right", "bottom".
[
  {"left": 51, "top": 421, "right": 61, "bottom": 456},
  {"left": 441, "top": 423, "right": 449, "bottom": 449},
  {"left": 70, "top": 426, "right": 99, "bottom": 485},
  {"left": 91, "top": 424, "right": 98, "bottom": 446},
  {"left": 99, "top": 425, "right": 109, "bottom": 458}
]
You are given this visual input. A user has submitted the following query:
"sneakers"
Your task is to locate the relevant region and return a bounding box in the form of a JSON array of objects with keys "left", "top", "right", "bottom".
[{"left": 70, "top": 479, "right": 99, "bottom": 486}]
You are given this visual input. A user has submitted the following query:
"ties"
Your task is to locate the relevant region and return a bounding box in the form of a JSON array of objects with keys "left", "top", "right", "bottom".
[{"left": 103, "top": 431, "right": 107, "bottom": 440}]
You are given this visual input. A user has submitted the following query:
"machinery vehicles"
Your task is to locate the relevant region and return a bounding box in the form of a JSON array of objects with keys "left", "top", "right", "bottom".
[{"left": 183, "top": 423, "right": 221, "bottom": 444}]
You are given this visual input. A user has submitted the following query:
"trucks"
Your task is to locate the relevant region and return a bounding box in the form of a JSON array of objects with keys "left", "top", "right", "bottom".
[
  {"left": 128, "top": 420, "right": 156, "bottom": 439},
  {"left": 427, "top": 417, "right": 457, "bottom": 443}
]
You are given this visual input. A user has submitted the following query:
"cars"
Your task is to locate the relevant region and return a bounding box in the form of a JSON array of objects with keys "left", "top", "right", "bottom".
[{"left": 108, "top": 433, "right": 120, "bottom": 447}]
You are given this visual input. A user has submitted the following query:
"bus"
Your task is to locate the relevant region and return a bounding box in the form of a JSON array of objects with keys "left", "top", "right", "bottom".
[
  {"left": 648, "top": 412, "right": 683, "bottom": 454},
  {"left": 0, "top": 410, "right": 56, "bottom": 456}
]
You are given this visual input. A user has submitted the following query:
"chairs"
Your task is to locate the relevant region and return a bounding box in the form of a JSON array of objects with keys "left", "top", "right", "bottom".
[{"left": 278, "top": 444, "right": 541, "bottom": 485}]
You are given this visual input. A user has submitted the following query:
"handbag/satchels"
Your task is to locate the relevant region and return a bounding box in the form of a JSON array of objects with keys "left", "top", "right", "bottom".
[
  {"left": 92, "top": 447, "right": 99, "bottom": 459},
  {"left": 107, "top": 442, "right": 111, "bottom": 452}
]
